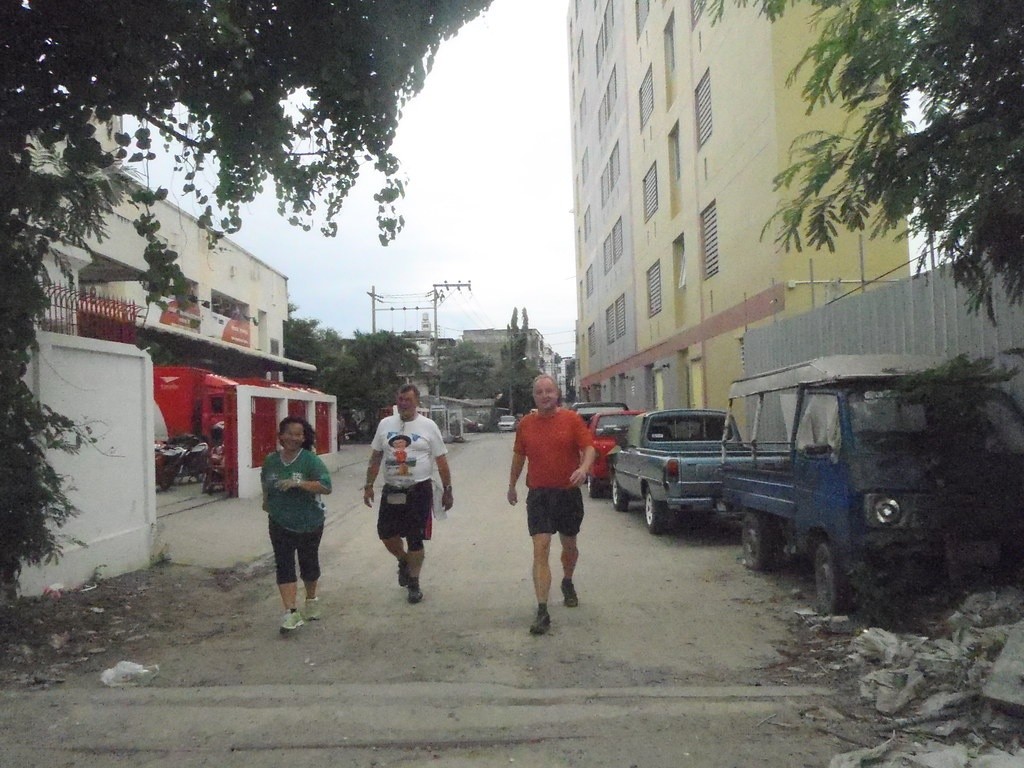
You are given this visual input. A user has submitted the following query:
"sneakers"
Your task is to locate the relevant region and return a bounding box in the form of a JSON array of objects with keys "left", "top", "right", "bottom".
[
  {"left": 279, "top": 611, "right": 305, "bottom": 633},
  {"left": 398, "top": 553, "right": 409, "bottom": 587},
  {"left": 561, "top": 581, "right": 578, "bottom": 607},
  {"left": 304, "top": 607, "right": 322, "bottom": 620},
  {"left": 530, "top": 612, "right": 550, "bottom": 634},
  {"left": 408, "top": 581, "right": 423, "bottom": 602}
]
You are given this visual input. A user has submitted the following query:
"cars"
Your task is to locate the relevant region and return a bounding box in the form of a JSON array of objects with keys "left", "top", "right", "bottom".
[
  {"left": 585, "top": 409, "right": 646, "bottom": 497},
  {"left": 497, "top": 415, "right": 517, "bottom": 432},
  {"left": 569, "top": 402, "right": 629, "bottom": 423},
  {"left": 463, "top": 417, "right": 478, "bottom": 433}
]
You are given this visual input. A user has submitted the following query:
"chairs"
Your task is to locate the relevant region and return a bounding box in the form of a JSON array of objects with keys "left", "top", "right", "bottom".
[
  {"left": 699, "top": 424, "right": 724, "bottom": 440},
  {"left": 649, "top": 426, "right": 674, "bottom": 441}
]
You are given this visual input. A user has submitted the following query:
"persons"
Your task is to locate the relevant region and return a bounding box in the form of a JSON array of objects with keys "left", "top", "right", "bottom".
[
  {"left": 260, "top": 415, "right": 332, "bottom": 634},
  {"left": 507, "top": 374, "right": 595, "bottom": 635},
  {"left": 362, "top": 384, "right": 453, "bottom": 603}
]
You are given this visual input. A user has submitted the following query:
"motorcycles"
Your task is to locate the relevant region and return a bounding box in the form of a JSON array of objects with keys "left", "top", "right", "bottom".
[
  {"left": 154, "top": 440, "right": 173, "bottom": 485},
  {"left": 161, "top": 442, "right": 209, "bottom": 490}
]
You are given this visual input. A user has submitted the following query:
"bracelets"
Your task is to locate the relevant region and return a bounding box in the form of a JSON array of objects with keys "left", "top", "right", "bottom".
[
  {"left": 295, "top": 477, "right": 300, "bottom": 488},
  {"left": 509, "top": 483, "right": 516, "bottom": 487},
  {"left": 363, "top": 482, "right": 374, "bottom": 491}
]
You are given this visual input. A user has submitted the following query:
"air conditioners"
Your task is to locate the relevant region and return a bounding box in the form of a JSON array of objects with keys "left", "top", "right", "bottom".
[{"left": 85, "top": 286, "right": 101, "bottom": 303}]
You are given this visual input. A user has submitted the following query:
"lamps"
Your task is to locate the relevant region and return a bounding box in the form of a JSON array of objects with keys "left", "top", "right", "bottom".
[{"left": 187, "top": 295, "right": 259, "bottom": 326}]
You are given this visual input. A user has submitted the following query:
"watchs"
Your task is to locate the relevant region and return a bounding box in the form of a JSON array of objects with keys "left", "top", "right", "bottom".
[{"left": 442, "top": 485, "right": 452, "bottom": 492}]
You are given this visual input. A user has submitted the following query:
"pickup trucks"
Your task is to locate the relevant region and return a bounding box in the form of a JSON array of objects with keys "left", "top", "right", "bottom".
[
  {"left": 611, "top": 407, "right": 790, "bottom": 533},
  {"left": 721, "top": 353, "right": 1023, "bottom": 621}
]
